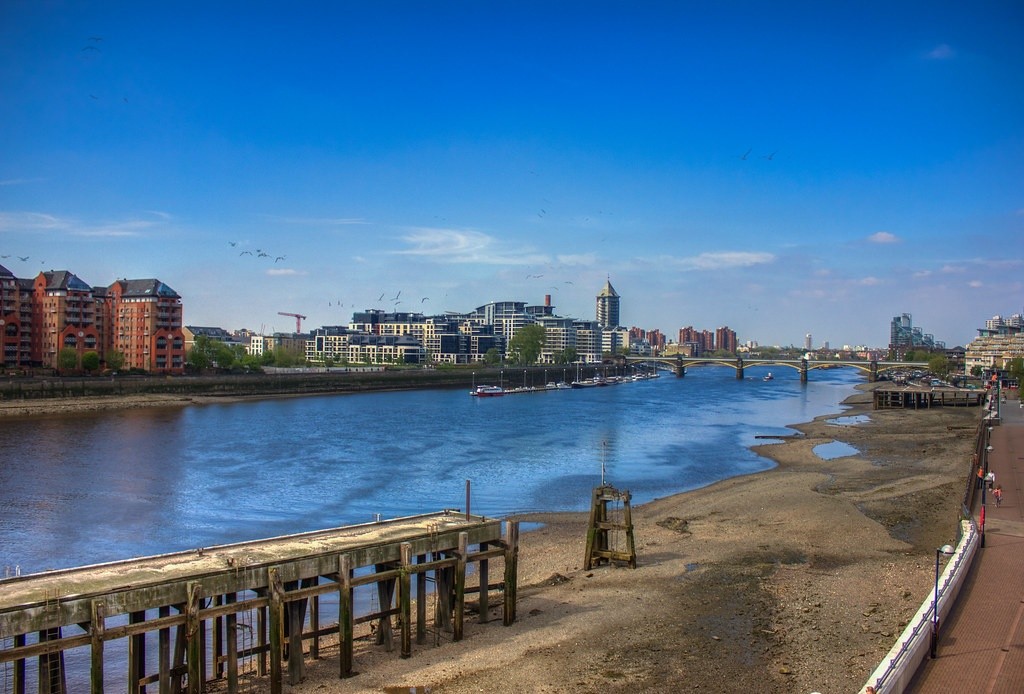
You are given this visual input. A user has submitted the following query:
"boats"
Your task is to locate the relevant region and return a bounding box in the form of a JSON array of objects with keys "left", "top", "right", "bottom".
[
  {"left": 477, "top": 386, "right": 504, "bottom": 397},
  {"left": 546, "top": 373, "right": 660, "bottom": 387}
]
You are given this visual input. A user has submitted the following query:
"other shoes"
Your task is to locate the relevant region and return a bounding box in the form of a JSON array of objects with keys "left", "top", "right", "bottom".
[
  {"left": 999, "top": 506, "right": 1000, "bottom": 508},
  {"left": 995, "top": 505, "right": 998, "bottom": 507}
]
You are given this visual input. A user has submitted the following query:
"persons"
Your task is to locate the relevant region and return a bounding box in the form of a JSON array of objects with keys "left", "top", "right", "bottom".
[
  {"left": 977, "top": 464, "right": 1003, "bottom": 508},
  {"left": 1001, "top": 391, "right": 1007, "bottom": 403}
]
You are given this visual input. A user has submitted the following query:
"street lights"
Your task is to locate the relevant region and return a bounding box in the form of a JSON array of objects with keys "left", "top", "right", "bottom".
[
  {"left": 981, "top": 406, "right": 995, "bottom": 548},
  {"left": 931, "top": 545, "right": 957, "bottom": 659}
]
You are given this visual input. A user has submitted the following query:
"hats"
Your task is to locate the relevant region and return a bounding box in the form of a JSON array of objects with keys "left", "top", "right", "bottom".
[{"left": 995, "top": 484, "right": 1002, "bottom": 489}]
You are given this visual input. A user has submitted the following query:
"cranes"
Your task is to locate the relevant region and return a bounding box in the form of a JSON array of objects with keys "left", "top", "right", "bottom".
[{"left": 277, "top": 311, "right": 306, "bottom": 334}]
